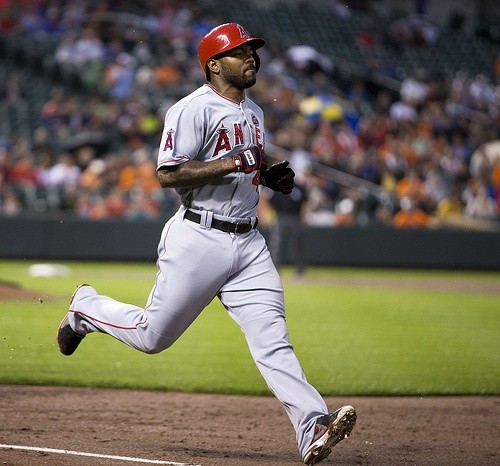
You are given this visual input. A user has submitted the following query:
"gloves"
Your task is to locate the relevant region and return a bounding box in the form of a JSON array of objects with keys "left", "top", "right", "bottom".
[
  {"left": 259, "top": 160, "right": 296, "bottom": 195},
  {"left": 232, "top": 143, "right": 265, "bottom": 173}
]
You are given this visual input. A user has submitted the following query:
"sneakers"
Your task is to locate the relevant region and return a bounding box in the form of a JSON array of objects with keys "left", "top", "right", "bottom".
[
  {"left": 55, "top": 285, "right": 94, "bottom": 357},
  {"left": 302, "top": 405, "right": 358, "bottom": 465}
]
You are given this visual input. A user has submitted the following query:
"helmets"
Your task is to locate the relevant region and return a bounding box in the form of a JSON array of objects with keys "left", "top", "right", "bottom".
[{"left": 197, "top": 22, "right": 266, "bottom": 82}]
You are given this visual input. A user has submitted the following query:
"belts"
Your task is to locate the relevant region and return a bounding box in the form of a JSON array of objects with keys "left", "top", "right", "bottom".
[{"left": 185, "top": 209, "right": 258, "bottom": 234}]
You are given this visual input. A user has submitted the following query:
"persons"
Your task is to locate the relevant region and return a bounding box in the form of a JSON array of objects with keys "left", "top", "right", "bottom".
[
  {"left": 55, "top": 22, "right": 357, "bottom": 466},
  {"left": 0, "top": 0, "right": 500, "bottom": 230}
]
